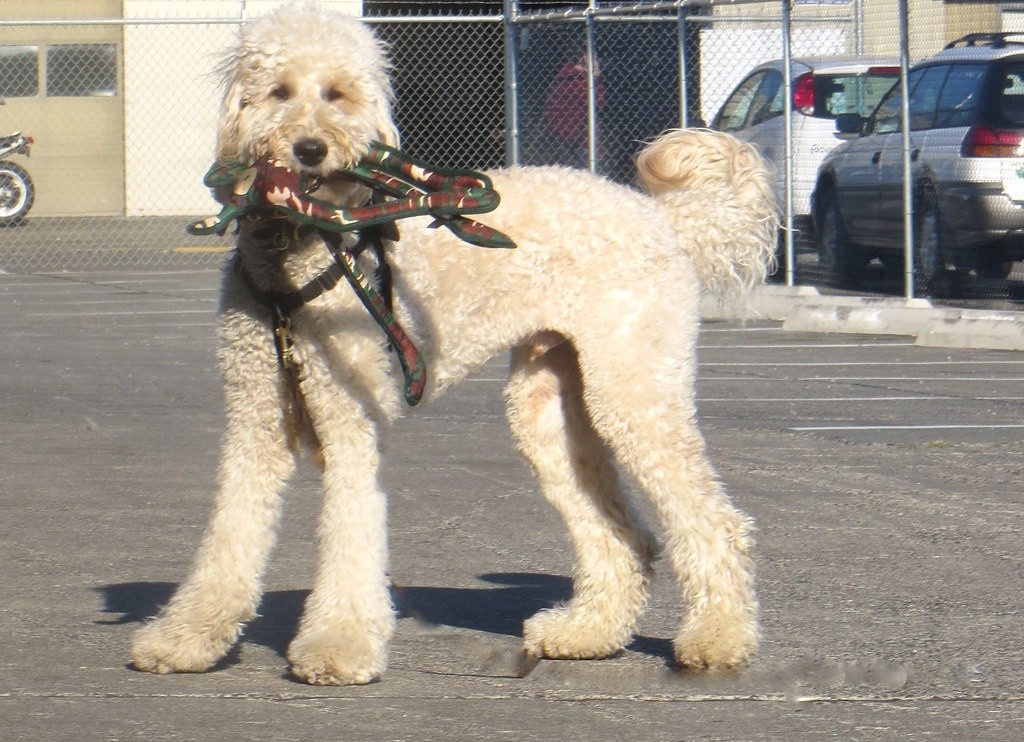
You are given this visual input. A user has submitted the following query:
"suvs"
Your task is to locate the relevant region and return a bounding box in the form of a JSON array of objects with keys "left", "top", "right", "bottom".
[{"left": 810, "top": 31, "right": 1024, "bottom": 298}]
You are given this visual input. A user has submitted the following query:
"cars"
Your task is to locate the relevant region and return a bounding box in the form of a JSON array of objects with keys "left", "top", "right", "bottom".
[{"left": 688, "top": 57, "right": 913, "bottom": 285}]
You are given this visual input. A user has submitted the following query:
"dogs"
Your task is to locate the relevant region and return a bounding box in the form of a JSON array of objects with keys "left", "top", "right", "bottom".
[{"left": 126, "top": 1, "right": 800, "bottom": 685}]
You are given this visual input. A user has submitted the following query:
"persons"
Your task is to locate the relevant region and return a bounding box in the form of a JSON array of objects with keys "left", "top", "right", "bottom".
[{"left": 546, "top": 44, "right": 605, "bottom": 172}]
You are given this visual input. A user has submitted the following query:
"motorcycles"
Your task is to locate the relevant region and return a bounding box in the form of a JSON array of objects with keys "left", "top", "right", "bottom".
[{"left": 0, "top": 131, "right": 36, "bottom": 225}]
